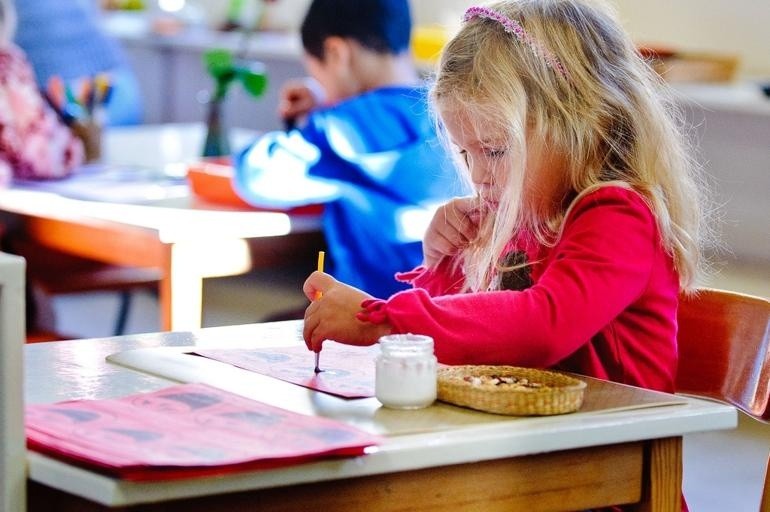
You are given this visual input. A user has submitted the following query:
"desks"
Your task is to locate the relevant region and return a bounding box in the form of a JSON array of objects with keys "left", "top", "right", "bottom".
[
  {"left": 3, "top": 195, "right": 322, "bottom": 331},
  {"left": 22, "top": 313, "right": 738, "bottom": 512}
]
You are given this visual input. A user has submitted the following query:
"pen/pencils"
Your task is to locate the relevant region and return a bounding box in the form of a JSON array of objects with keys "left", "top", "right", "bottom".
[{"left": 40, "top": 73, "right": 114, "bottom": 128}]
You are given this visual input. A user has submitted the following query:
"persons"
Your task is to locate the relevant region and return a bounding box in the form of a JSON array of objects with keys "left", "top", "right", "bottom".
[
  {"left": 8, "top": 0, "right": 146, "bottom": 127},
  {"left": 0, "top": 0, "right": 89, "bottom": 333},
  {"left": 301, "top": 0, "right": 701, "bottom": 394},
  {"left": 230, "top": 1, "right": 478, "bottom": 323}
]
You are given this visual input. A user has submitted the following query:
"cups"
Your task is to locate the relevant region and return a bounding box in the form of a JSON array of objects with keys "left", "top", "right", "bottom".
[{"left": 73, "top": 122, "right": 106, "bottom": 162}]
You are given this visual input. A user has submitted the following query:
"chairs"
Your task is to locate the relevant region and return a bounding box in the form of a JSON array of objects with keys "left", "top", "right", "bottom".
[{"left": 681, "top": 284, "right": 770, "bottom": 511}]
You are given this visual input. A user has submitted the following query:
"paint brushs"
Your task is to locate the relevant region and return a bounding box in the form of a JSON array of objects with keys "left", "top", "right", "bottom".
[{"left": 314, "top": 251, "right": 325, "bottom": 373}]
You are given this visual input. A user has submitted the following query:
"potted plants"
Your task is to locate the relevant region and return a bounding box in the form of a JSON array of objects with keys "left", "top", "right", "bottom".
[{"left": 201, "top": 2, "right": 269, "bottom": 154}]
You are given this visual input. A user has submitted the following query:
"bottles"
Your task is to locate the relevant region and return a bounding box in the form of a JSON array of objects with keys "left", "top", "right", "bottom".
[{"left": 374, "top": 334, "right": 439, "bottom": 411}]
